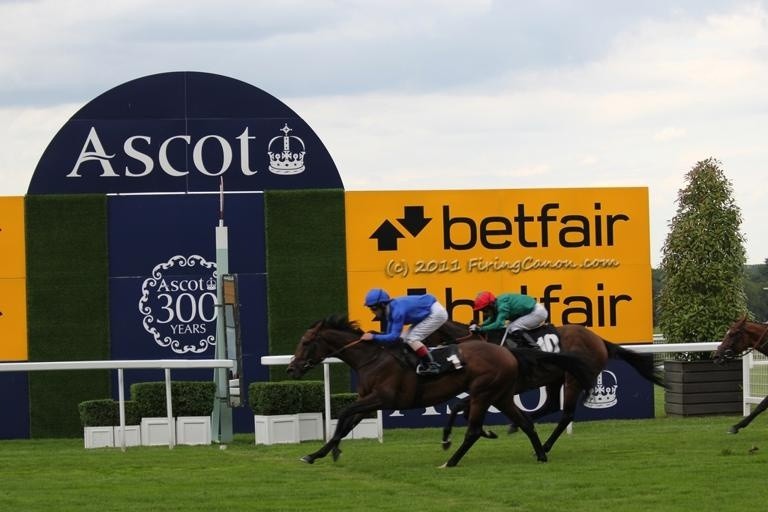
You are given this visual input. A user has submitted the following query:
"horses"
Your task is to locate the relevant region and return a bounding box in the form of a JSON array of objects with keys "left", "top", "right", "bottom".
[
  {"left": 710, "top": 312, "right": 768, "bottom": 435},
  {"left": 422, "top": 319, "right": 672, "bottom": 457},
  {"left": 284, "top": 314, "right": 587, "bottom": 469}
]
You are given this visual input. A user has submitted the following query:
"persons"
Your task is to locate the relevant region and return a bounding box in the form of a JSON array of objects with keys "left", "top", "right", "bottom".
[
  {"left": 469, "top": 290, "right": 549, "bottom": 350},
  {"left": 359, "top": 288, "right": 449, "bottom": 372}
]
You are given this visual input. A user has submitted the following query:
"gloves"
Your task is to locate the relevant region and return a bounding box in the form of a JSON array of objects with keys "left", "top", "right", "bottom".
[{"left": 469, "top": 324, "right": 481, "bottom": 333}]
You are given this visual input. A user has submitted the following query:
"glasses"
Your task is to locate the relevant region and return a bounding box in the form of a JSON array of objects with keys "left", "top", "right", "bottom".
[{"left": 372, "top": 306, "right": 377, "bottom": 311}]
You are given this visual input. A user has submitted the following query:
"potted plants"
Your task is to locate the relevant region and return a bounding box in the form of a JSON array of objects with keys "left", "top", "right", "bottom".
[
  {"left": 351, "top": 393, "right": 386, "bottom": 444},
  {"left": 246, "top": 376, "right": 305, "bottom": 449},
  {"left": 170, "top": 379, "right": 216, "bottom": 448},
  {"left": 78, "top": 396, "right": 115, "bottom": 449},
  {"left": 656, "top": 156, "right": 752, "bottom": 420},
  {"left": 327, "top": 390, "right": 354, "bottom": 446},
  {"left": 112, "top": 400, "right": 144, "bottom": 450},
  {"left": 129, "top": 380, "right": 176, "bottom": 448},
  {"left": 284, "top": 377, "right": 327, "bottom": 442}
]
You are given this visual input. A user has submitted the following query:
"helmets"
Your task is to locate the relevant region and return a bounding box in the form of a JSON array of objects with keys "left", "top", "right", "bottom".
[
  {"left": 473, "top": 292, "right": 496, "bottom": 311},
  {"left": 366, "top": 288, "right": 389, "bottom": 306}
]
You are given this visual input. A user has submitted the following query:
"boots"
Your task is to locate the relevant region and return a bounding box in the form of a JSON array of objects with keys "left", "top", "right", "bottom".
[
  {"left": 419, "top": 353, "right": 441, "bottom": 374},
  {"left": 513, "top": 330, "right": 540, "bottom": 351}
]
